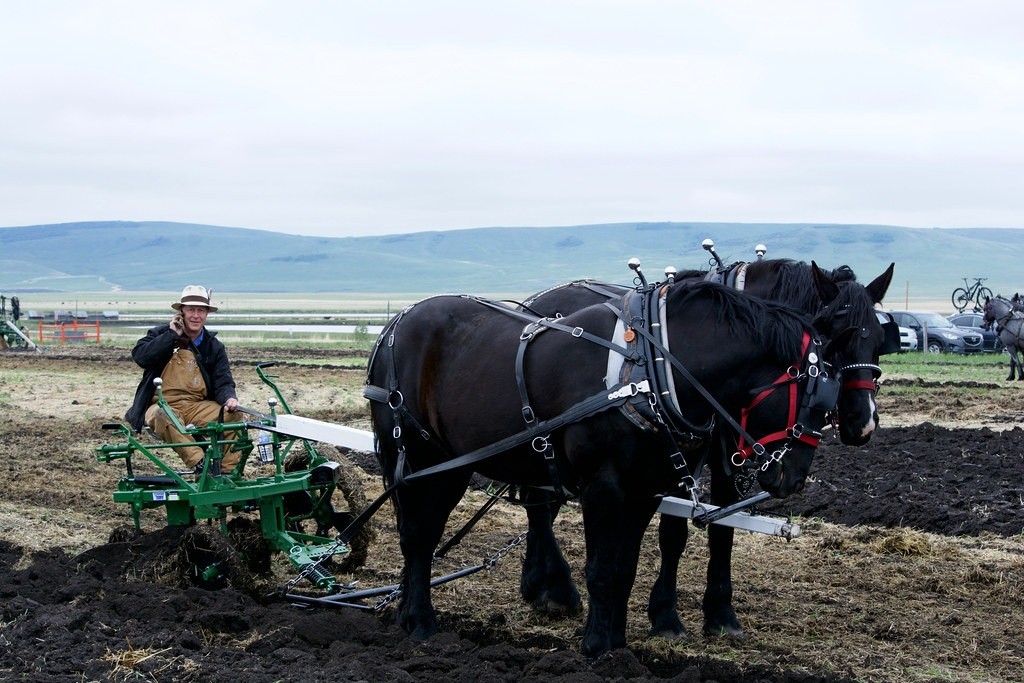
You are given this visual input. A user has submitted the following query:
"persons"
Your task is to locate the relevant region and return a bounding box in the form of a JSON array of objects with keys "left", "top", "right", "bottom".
[{"left": 124, "top": 285, "right": 244, "bottom": 484}]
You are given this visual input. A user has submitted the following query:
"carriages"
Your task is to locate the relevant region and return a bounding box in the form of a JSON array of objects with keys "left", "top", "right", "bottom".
[{"left": 94, "top": 235, "right": 896, "bottom": 657}]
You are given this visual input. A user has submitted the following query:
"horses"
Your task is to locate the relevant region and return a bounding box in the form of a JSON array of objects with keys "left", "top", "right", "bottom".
[
  {"left": 366, "top": 281, "right": 860, "bottom": 660},
  {"left": 510, "top": 258, "right": 895, "bottom": 635},
  {"left": 983, "top": 293, "right": 1024, "bottom": 381}
]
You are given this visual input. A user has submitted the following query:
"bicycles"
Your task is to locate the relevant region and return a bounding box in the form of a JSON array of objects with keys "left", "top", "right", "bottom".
[{"left": 951, "top": 277, "right": 993, "bottom": 309}]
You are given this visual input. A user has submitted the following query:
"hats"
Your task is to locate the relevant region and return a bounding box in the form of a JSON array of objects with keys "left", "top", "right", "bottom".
[{"left": 171, "top": 286, "right": 218, "bottom": 312}]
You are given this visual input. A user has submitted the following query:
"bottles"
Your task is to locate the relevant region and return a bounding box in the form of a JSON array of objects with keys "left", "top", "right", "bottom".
[{"left": 258, "top": 428, "right": 274, "bottom": 462}]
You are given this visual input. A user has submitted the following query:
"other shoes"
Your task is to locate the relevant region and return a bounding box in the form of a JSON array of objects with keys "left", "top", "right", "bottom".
[{"left": 193, "top": 457, "right": 212, "bottom": 483}]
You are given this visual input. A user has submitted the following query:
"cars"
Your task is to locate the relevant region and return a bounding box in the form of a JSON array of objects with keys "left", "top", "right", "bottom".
[
  {"left": 946, "top": 313, "right": 1009, "bottom": 353},
  {"left": 890, "top": 310, "right": 983, "bottom": 354},
  {"left": 875, "top": 312, "right": 918, "bottom": 352}
]
